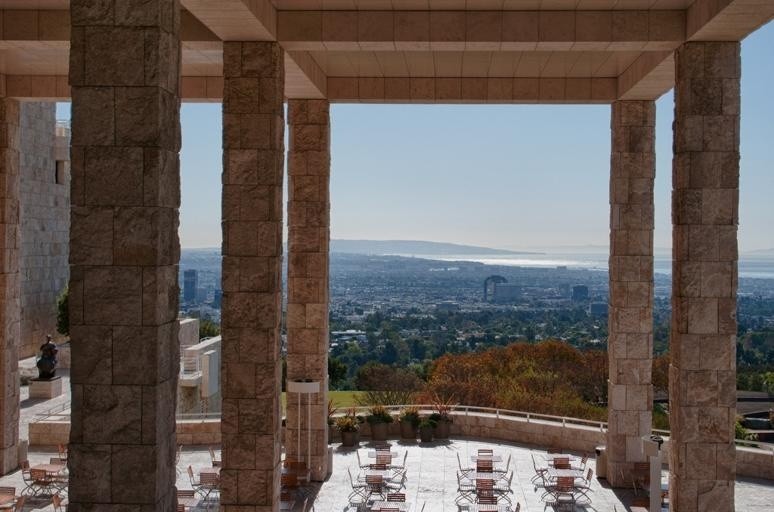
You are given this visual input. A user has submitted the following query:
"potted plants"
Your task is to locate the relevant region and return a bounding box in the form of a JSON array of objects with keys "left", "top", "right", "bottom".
[{"left": 328, "top": 391, "right": 455, "bottom": 445}]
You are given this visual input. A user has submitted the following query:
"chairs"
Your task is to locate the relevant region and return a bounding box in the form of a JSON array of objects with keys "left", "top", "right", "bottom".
[
  {"left": 348, "top": 445, "right": 593, "bottom": 512},
  {"left": 0, "top": 443, "right": 68, "bottom": 512},
  {"left": 621, "top": 463, "right": 668, "bottom": 507},
  {"left": 177, "top": 446, "right": 219, "bottom": 512}
]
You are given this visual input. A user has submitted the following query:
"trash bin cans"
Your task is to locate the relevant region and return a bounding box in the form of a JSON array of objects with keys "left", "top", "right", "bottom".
[
  {"left": 595, "top": 445, "right": 607, "bottom": 478},
  {"left": 328, "top": 445, "right": 333, "bottom": 473}
]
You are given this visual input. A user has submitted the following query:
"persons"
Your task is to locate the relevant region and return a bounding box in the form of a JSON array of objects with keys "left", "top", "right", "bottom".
[{"left": 39, "top": 334, "right": 57, "bottom": 363}]
